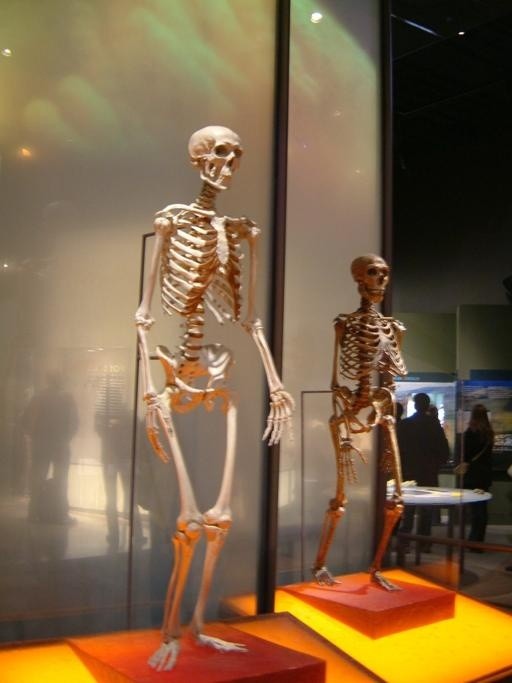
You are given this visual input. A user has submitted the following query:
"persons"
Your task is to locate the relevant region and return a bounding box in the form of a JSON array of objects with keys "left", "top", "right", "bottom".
[
  {"left": 387, "top": 391, "right": 449, "bottom": 554},
  {"left": 391, "top": 401, "right": 447, "bottom": 534},
  {"left": 23, "top": 366, "right": 82, "bottom": 527},
  {"left": 452, "top": 404, "right": 496, "bottom": 554},
  {"left": 95, "top": 387, "right": 149, "bottom": 547}
]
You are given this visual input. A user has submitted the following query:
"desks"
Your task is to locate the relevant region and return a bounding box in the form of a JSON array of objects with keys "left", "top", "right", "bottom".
[{"left": 387, "top": 486, "right": 493, "bottom": 572}]
